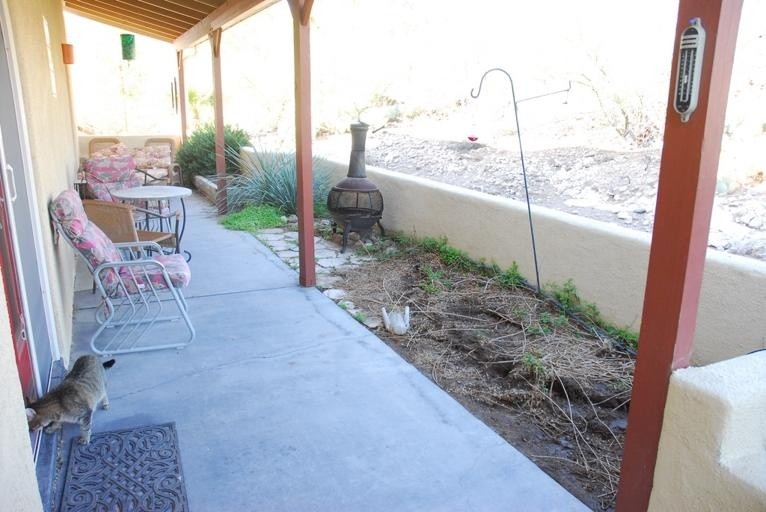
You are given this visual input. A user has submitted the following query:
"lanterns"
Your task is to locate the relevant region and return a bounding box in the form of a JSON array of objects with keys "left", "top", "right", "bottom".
[{"left": 120, "top": 33, "right": 136, "bottom": 68}]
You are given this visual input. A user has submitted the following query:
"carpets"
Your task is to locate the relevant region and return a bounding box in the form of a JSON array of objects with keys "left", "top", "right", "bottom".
[{"left": 55, "top": 422, "right": 190, "bottom": 511}]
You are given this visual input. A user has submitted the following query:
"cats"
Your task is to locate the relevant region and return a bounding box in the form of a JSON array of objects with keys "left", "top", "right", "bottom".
[{"left": 24, "top": 354, "right": 116, "bottom": 446}]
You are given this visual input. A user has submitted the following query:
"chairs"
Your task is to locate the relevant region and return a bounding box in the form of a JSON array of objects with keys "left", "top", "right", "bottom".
[{"left": 48, "top": 138, "right": 196, "bottom": 356}]
